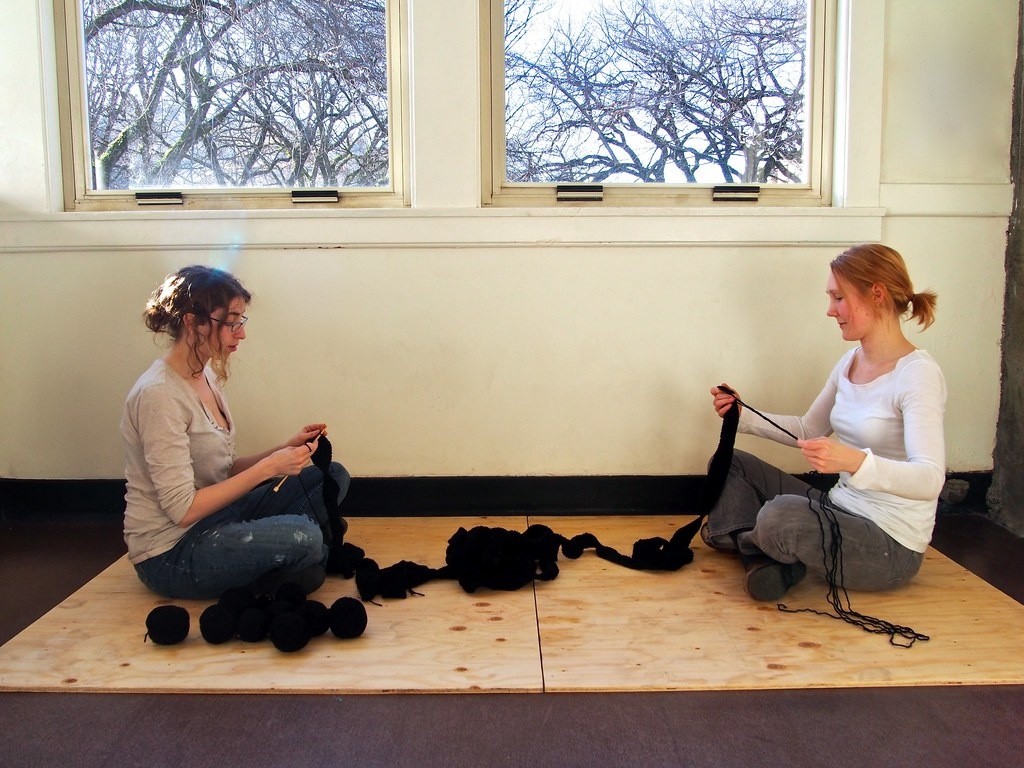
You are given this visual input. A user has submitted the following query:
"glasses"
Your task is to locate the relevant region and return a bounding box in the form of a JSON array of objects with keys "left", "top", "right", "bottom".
[{"left": 208, "top": 315, "right": 248, "bottom": 332}]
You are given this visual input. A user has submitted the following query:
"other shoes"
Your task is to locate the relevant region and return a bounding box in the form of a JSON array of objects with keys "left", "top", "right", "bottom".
[
  {"left": 744, "top": 560, "right": 808, "bottom": 602},
  {"left": 701, "top": 522, "right": 738, "bottom": 552}
]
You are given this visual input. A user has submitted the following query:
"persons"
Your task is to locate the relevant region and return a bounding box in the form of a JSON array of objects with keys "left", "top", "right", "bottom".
[
  {"left": 121, "top": 266, "right": 350, "bottom": 596},
  {"left": 700, "top": 245, "right": 948, "bottom": 603}
]
